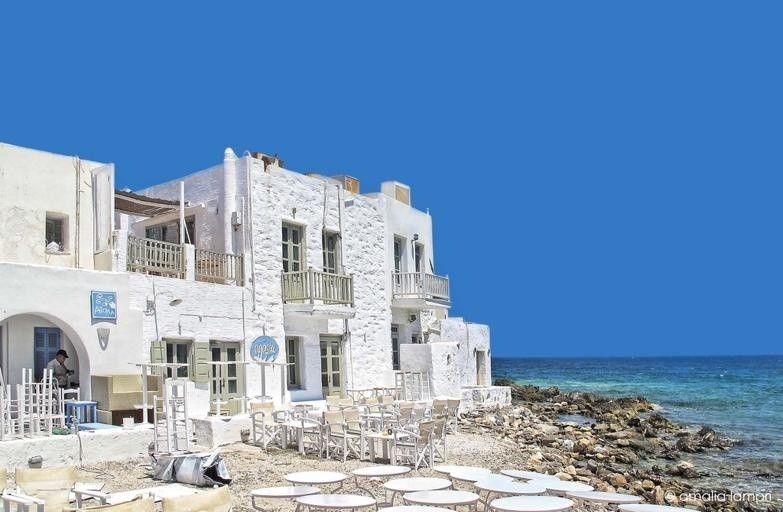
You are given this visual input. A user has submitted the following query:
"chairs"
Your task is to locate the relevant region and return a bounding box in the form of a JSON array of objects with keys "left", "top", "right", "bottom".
[
  {"left": 250, "top": 396, "right": 460, "bottom": 470},
  {"left": 0, "top": 464, "right": 233, "bottom": 512}
]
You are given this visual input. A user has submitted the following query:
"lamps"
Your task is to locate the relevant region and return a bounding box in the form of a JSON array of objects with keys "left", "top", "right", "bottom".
[
  {"left": 97, "top": 322, "right": 110, "bottom": 337},
  {"left": 411, "top": 234, "right": 418, "bottom": 243},
  {"left": 410, "top": 315, "right": 416, "bottom": 322}
]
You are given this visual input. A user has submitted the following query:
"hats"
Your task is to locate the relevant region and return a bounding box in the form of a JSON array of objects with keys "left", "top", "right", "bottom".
[{"left": 55, "top": 349, "right": 69, "bottom": 358}]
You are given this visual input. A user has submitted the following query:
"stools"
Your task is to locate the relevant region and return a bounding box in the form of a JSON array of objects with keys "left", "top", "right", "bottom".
[{"left": 66, "top": 401, "right": 97, "bottom": 423}]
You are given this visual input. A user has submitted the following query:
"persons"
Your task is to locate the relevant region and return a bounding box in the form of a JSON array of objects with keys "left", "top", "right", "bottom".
[{"left": 47, "top": 349, "right": 73, "bottom": 425}]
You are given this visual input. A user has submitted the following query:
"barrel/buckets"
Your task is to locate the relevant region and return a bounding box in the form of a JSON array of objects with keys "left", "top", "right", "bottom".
[{"left": 123, "top": 418, "right": 134, "bottom": 430}]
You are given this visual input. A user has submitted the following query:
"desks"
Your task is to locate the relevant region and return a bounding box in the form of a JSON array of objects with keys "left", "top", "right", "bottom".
[{"left": 251, "top": 465, "right": 701, "bottom": 512}]
